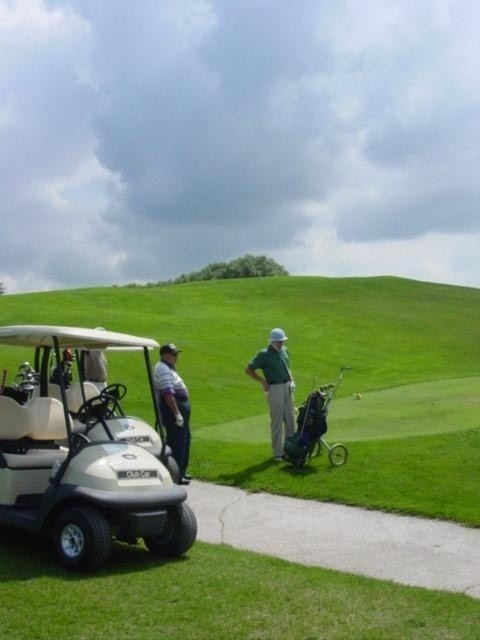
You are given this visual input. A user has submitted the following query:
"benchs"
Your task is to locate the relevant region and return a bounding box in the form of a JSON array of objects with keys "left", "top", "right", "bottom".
[{"left": 0, "top": 382, "right": 110, "bottom": 469}]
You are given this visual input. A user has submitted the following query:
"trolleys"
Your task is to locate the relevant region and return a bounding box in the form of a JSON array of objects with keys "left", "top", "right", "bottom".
[{"left": 281, "top": 365, "right": 355, "bottom": 468}]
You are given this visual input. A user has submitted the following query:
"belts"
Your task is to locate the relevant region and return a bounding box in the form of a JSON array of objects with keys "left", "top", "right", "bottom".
[
  {"left": 270, "top": 380, "right": 288, "bottom": 384},
  {"left": 86, "top": 377, "right": 105, "bottom": 383}
]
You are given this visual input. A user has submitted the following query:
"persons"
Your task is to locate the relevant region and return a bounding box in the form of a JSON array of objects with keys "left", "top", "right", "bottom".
[
  {"left": 82, "top": 327, "right": 107, "bottom": 395},
  {"left": 154, "top": 343, "right": 193, "bottom": 486},
  {"left": 243, "top": 328, "right": 298, "bottom": 463}
]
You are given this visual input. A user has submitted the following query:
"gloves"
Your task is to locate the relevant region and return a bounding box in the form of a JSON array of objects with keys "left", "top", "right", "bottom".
[
  {"left": 289, "top": 381, "right": 296, "bottom": 392},
  {"left": 175, "top": 412, "right": 184, "bottom": 427}
]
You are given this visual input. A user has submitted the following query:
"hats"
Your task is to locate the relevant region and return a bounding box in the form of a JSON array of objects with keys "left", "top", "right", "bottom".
[
  {"left": 270, "top": 328, "right": 288, "bottom": 342},
  {"left": 160, "top": 343, "right": 183, "bottom": 355}
]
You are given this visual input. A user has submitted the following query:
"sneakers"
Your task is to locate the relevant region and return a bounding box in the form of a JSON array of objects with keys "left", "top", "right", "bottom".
[
  {"left": 274, "top": 455, "right": 282, "bottom": 461},
  {"left": 179, "top": 474, "right": 193, "bottom": 485}
]
